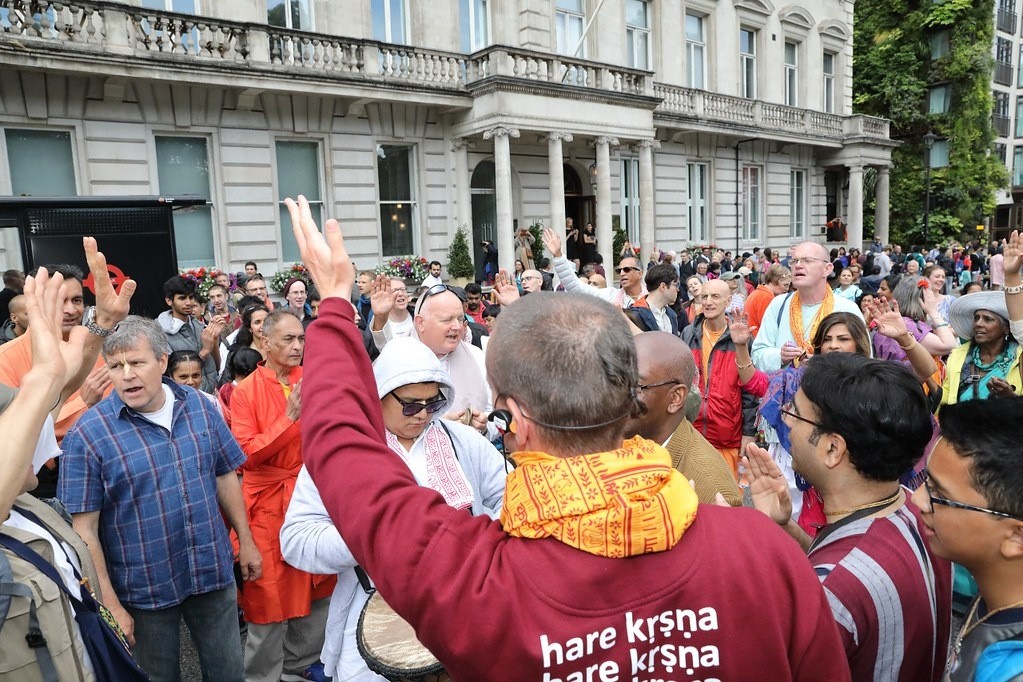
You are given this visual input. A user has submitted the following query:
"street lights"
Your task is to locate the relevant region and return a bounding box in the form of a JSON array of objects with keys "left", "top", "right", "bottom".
[{"left": 921, "top": 129, "right": 936, "bottom": 246}]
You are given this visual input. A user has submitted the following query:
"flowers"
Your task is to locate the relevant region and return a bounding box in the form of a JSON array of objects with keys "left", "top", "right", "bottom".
[
  {"left": 269, "top": 263, "right": 311, "bottom": 293},
  {"left": 374, "top": 254, "right": 429, "bottom": 282},
  {"left": 695, "top": 244, "right": 717, "bottom": 257},
  {"left": 178, "top": 266, "right": 237, "bottom": 299}
]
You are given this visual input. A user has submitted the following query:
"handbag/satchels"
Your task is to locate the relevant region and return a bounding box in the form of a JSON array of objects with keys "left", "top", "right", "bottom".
[{"left": 0, "top": 504, "right": 152, "bottom": 682}]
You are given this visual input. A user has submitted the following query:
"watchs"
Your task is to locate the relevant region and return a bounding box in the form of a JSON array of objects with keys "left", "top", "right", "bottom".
[{"left": 81, "top": 305, "right": 118, "bottom": 338}]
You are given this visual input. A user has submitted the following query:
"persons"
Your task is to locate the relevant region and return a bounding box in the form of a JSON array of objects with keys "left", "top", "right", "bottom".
[{"left": 0, "top": 194, "right": 1023, "bottom": 682}]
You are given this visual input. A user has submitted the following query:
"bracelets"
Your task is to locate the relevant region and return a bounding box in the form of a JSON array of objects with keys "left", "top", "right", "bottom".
[
  {"left": 934, "top": 321, "right": 948, "bottom": 329},
  {"left": 1003, "top": 280, "right": 1023, "bottom": 294},
  {"left": 735, "top": 357, "right": 752, "bottom": 370},
  {"left": 900, "top": 332, "right": 917, "bottom": 351}
]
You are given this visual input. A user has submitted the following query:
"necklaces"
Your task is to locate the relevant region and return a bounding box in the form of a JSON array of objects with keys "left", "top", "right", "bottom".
[
  {"left": 822, "top": 487, "right": 901, "bottom": 516},
  {"left": 955, "top": 593, "right": 1023, "bottom": 655}
]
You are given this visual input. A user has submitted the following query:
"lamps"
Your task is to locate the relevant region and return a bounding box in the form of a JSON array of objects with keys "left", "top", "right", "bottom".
[{"left": 589, "top": 162, "right": 596, "bottom": 190}]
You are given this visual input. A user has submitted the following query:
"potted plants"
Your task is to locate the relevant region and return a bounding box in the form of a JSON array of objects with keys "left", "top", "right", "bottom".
[
  {"left": 613, "top": 229, "right": 630, "bottom": 280},
  {"left": 446, "top": 227, "right": 476, "bottom": 289}
]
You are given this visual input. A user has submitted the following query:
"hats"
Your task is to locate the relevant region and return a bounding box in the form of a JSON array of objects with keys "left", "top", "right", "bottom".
[
  {"left": 719, "top": 271, "right": 740, "bottom": 281},
  {"left": 284, "top": 276, "right": 308, "bottom": 300},
  {"left": 446, "top": 285, "right": 467, "bottom": 303},
  {"left": 947, "top": 290, "right": 1010, "bottom": 340},
  {"left": 738, "top": 266, "right": 753, "bottom": 277}
]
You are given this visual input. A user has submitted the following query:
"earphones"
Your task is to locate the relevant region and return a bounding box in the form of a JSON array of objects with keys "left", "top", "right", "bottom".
[{"left": 489, "top": 409, "right": 513, "bottom": 435}]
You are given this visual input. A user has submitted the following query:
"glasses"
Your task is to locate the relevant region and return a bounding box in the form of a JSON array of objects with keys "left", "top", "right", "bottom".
[
  {"left": 390, "top": 389, "right": 448, "bottom": 417},
  {"left": 670, "top": 282, "right": 680, "bottom": 288},
  {"left": 433, "top": 269, "right": 440, "bottom": 271},
  {"left": 289, "top": 290, "right": 309, "bottom": 296},
  {"left": 779, "top": 401, "right": 831, "bottom": 431},
  {"left": 418, "top": 284, "right": 452, "bottom": 314},
  {"left": 521, "top": 276, "right": 538, "bottom": 281},
  {"left": 468, "top": 297, "right": 479, "bottom": 300},
  {"left": 248, "top": 305, "right": 266, "bottom": 311},
  {"left": 787, "top": 256, "right": 832, "bottom": 267},
  {"left": 616, "top": 265, "right": 641, "bottom": 276},
  {"left": 924, "top": 466, "right": 1023, "bottom": 521},
  {"left": 637, "top": 379, "right": 681, "bottom": 394},
  {"left": 393, "top": 287, "right": 407, "bottom": 293}
]
